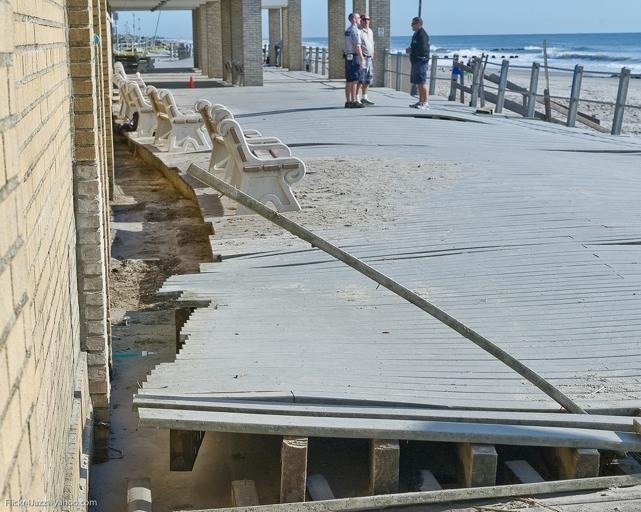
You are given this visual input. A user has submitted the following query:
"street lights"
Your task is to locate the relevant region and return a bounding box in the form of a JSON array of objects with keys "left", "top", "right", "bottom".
[{"left": 112, "top": 12, "right": 144, "bottom": 43}]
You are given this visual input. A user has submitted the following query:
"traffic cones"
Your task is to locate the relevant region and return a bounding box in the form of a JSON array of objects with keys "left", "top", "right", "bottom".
[{"left": 188, "top": 76, "right": 195, "bottom": 88}]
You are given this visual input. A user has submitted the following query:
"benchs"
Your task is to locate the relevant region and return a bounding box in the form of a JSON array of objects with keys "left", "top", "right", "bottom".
[{"left": 108, "top": 55, "right": 311, "bottom": 217}]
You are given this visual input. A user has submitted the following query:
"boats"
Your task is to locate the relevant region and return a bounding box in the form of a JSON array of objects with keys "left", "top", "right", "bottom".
[{"left": 113, "top": 38, "right": 178, "bottom": 63}]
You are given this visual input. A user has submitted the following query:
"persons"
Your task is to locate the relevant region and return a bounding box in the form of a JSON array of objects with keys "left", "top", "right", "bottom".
[
  {"left": 406, "top": 17, "right": 430, "bottom": 109},
  {"left": 342, "top": 13, "right": 365, "bottom": 108},
  {"left": 356, "top": 14, "right": 375, "bottom": 105},
  {"left": 460, "top": 59, "right": 477, "bottom": 83}
]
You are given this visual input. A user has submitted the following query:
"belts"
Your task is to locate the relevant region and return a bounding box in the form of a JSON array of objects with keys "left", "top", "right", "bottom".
[{"left": 362, "top": 53, "right": 373, "bottom": 58}]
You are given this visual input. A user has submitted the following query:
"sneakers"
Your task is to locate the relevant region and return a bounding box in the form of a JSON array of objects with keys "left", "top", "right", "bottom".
[
  {"left": 344, "top": 101, "right": 350, "bottom": 108},
  {"left": 349, "top": 101, "right": 365, "bottom": 108},
  {"left": 360, "top": 98, "right": 375, "bottom": 105},
  {"left": 408, "top": 101, "right": 430, "bottom": 109}
]
